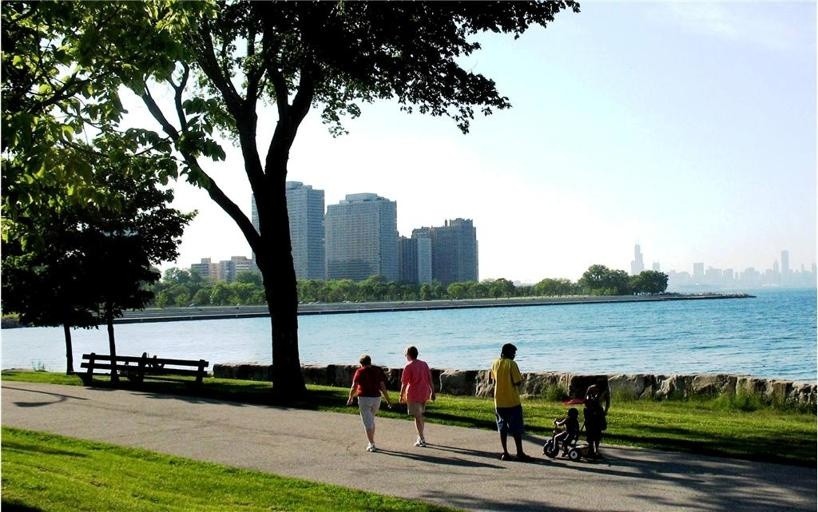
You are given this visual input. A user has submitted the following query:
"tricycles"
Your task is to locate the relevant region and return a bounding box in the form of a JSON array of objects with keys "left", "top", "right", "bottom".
[{"left": 543, "top": 417, "right": 594, "bottom": 460}]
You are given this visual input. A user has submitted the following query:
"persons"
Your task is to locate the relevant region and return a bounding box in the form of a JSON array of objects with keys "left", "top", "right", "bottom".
[
  {"left": 551, "top": 407, "right": 579, "bottom": 456},
  {"left": 346, "top": 355, "right": 392, "bottom": 453},
  {"left": 584, "top": 378, "right": 607, "bottom": 458},
  {"left": 400, "top": 346, "right": 436, "bottom": 447},
  {"left": 488, "top": 343, "right": 530, "bottom": 460}
]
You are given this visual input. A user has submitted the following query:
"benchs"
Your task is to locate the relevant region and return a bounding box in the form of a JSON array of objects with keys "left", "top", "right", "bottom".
[{"left": 71, "top": 352, "right": 209, "bottom": 396}]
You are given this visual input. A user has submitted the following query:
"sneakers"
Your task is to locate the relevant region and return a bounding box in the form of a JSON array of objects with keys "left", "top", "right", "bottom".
[
  {"left": 414, "top": 437, "right": 426, "bottom": 447},
  {"left": 500, "top": 453, "right": 533, "bottom": 461},
  {"left": 365, "top": 442, "right": 376, "bottom": 453}
]
